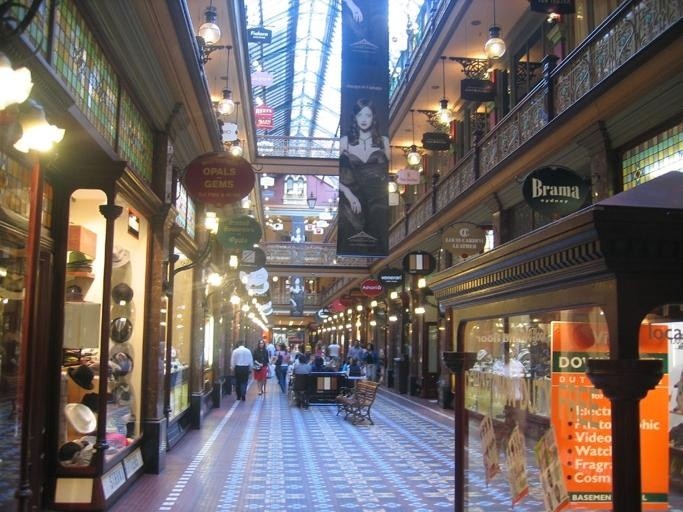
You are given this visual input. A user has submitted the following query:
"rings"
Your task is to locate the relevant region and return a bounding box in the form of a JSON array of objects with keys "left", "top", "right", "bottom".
[{"left": 352, "top": 200, "right": 357, "bottom": 205}]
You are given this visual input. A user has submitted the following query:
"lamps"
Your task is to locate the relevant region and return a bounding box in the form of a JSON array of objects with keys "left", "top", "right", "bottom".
[
  {"left": 219, "top": 46, "right": 234, "bottom": 117},
  {"left": 0, "top": 44, "right": 65, "bottom": 154},
  {"left": 173, "top": 211, "right": 220, "bottom": 279},
  {"left": 198, "top": 1, "right": 222, "bottom": 46},
  {"left": 230, "top": 139, "right": 243, "bottom": 156},
  {"left": 388, "top": 145, "right": 397, "bottom": 190},
  {"left": 413, "top": 280, "right": 437, "bottom": 315},
  {"left": 485, "top": 0, "right": 506, "bottom": 60},
  {"left": 436, "top": 56, "right": 452, "bottom": 125},
  {"left": 407, "top": 112, "right": 421, "bottom": 166}
]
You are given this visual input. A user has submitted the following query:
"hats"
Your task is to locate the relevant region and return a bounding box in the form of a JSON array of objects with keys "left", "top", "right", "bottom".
[{"left": 57, "top": 247, "right": 134, "bottom": 466}]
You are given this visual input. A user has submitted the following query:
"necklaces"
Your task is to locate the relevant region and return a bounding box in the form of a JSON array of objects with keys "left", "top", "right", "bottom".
[{"left": 357, "top": 132, "right": 374, "bottom": 151}]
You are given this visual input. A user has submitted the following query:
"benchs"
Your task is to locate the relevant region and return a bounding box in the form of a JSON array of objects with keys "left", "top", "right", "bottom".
[
  {"left": 337, "top": 380, "right": 382, "bottom": 425},
  {"left": 295, "top": 371, "right": 345, "bottom": 402}
]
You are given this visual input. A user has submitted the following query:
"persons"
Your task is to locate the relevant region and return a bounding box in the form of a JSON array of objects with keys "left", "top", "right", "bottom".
[
  {"left": 229, "top": 338, "right": 378, "bottom": 408},
  {"left": 290, "top": 278, "right": 305, "bottom": 309},
  {"left": 345, "top": 1, "right": 363, "bottom": 23},
  {"left": 340, "top": 97, "right": 390, "bottom": 216},
  {"left": 290, "top": 225, "right": 305, "bottom": 243}
]
